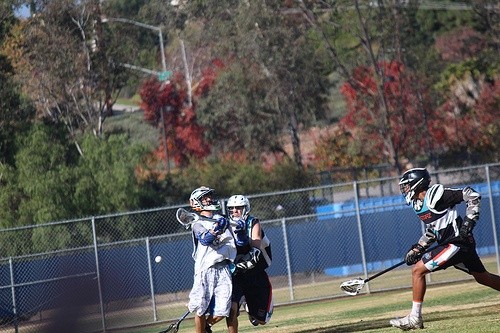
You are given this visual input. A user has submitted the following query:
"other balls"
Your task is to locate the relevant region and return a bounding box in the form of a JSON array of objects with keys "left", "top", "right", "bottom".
[{"left": 154, "top": 256, "right": 162, "bottom": 262}]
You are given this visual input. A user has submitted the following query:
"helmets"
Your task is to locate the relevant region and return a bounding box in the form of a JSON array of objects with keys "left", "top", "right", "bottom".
[
  {"left": 398, "top": 168, "right": 431, "bottom": 208},
  {"left": 226, "top": 194, "right": 250, "bottom": 225},
  {"left": 189, "top": 186, "right": 222, "bottom": 211}
]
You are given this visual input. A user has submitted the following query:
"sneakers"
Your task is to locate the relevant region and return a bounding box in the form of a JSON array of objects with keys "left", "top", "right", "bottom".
[
  {"left": 389, "top": 315, "right": 424, "bottom": 330},
  {"left": 243, "top": 301, "right": 258, "bottom": 326},
  {"left": 205, "top": 322, "right": 212, "bottom": 333}
]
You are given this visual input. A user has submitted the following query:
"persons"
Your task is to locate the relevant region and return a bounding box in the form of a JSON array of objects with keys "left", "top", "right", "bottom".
[
  {"left": 389, "top": 167, "right": 500, "bottom": 330},
  {"left": 205, "top": 194, "right": 273, "bottom": 326},
  {"left": 187, "top": 186, "right": 238, "bottom": 333}
]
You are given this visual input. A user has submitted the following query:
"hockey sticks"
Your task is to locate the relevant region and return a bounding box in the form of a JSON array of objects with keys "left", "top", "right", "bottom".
[
  {"left": 175, "top": 207, "right": 242, "bottom": 226},
  {"left": 156, "top": 311, "right": 191, "bottom": 333},
  {"left": 337, "top": 232, "right": 467, "bottom": 297}
]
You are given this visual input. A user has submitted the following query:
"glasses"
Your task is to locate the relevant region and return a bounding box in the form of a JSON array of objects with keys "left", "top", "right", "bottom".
[{"left": 228, "top": 206, "right": 246, "bottom": 211}]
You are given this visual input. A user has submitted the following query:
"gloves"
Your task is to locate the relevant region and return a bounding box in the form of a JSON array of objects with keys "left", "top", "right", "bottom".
[
  {"left": 233, "top": 219, "right": 247, "bottom": 234},
  {"left": 460, "top": 216, "right": 476, "bottom": 241},
  {"left": 404, "top": 243, "right": 425, "bottom": 266},
  {"left": 234, "top": 254, "right": 256, "bottom": 269},
  {"left": 211, "top": 217, "right": 230, "bottom": 235}
]
